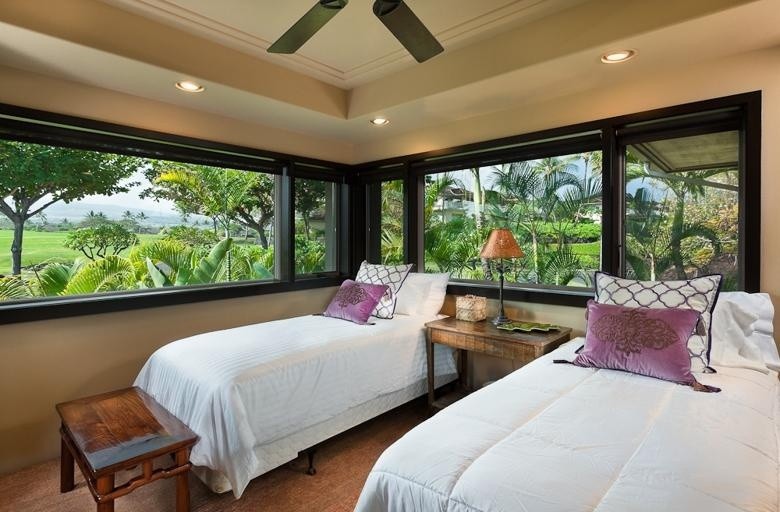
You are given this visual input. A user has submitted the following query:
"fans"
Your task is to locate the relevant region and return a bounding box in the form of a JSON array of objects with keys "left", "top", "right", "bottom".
[{"left": 264, "top": 0, "right": 445, "bottom": 63}]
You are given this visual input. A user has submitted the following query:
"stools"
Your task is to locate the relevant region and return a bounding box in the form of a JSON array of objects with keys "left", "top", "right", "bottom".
[{"left": 54, "top": 385, "right": 201, "bottom": 512}]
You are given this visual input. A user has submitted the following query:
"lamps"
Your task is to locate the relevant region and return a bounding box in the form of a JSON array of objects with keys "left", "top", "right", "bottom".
[{"left": 479, "top": 228, "right": 526, "bottom": 326}]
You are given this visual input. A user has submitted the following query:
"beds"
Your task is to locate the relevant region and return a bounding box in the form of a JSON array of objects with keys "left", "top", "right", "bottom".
[
  {"left": 372, "top": 334, "right": 780, "bottom": 512},
  {"left": 148, "top": 312, "right": 468, "bottom": 494}
]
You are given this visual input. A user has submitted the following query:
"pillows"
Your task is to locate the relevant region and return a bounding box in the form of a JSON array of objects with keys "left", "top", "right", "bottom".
[
  {"left": 322, "top": 260, "right": 451, "bottom": 327},
  {"left": 552, "top": 269, "right": 780, "bottom": 393}
]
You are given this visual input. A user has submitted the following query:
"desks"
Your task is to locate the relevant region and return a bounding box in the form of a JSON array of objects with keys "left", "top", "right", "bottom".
[{"left": 423, "top": 314, "right": 573, "bottom": 419}]
